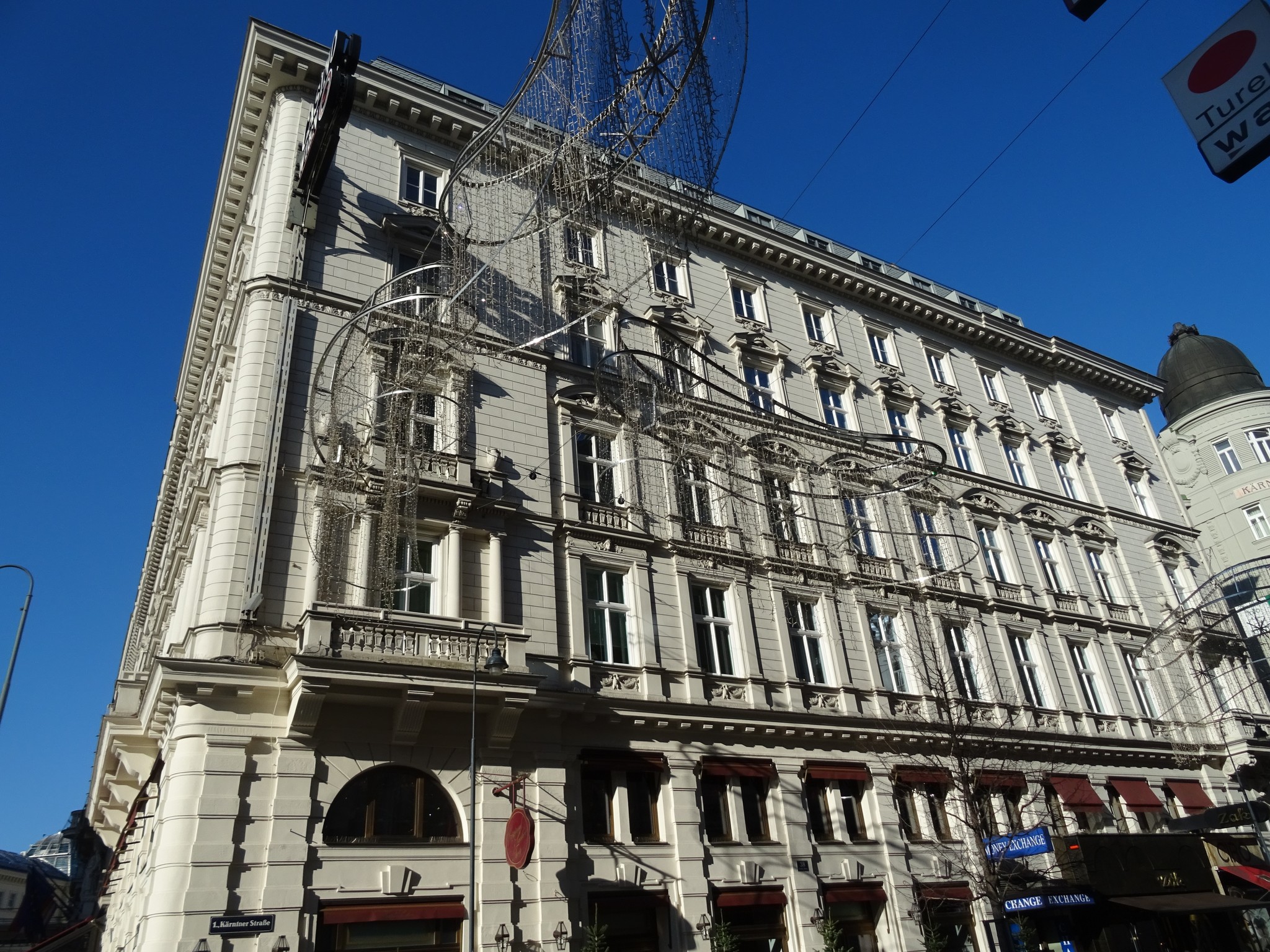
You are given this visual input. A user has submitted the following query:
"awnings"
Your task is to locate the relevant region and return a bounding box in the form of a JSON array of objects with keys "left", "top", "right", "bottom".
[
  {"left": 1112, "top": 863, "right": 1270, "bottom": 918},
  {"left": 25, "top": 915, "right": 99, "bottom": 952}
]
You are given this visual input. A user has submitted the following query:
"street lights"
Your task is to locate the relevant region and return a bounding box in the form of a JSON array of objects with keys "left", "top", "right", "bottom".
[
  {"left": 1218, "top": 708, "right": 1270, "bottom": 865},
  {"left": 468, "top": 622, "right": 510, "bottom": 951}
]
